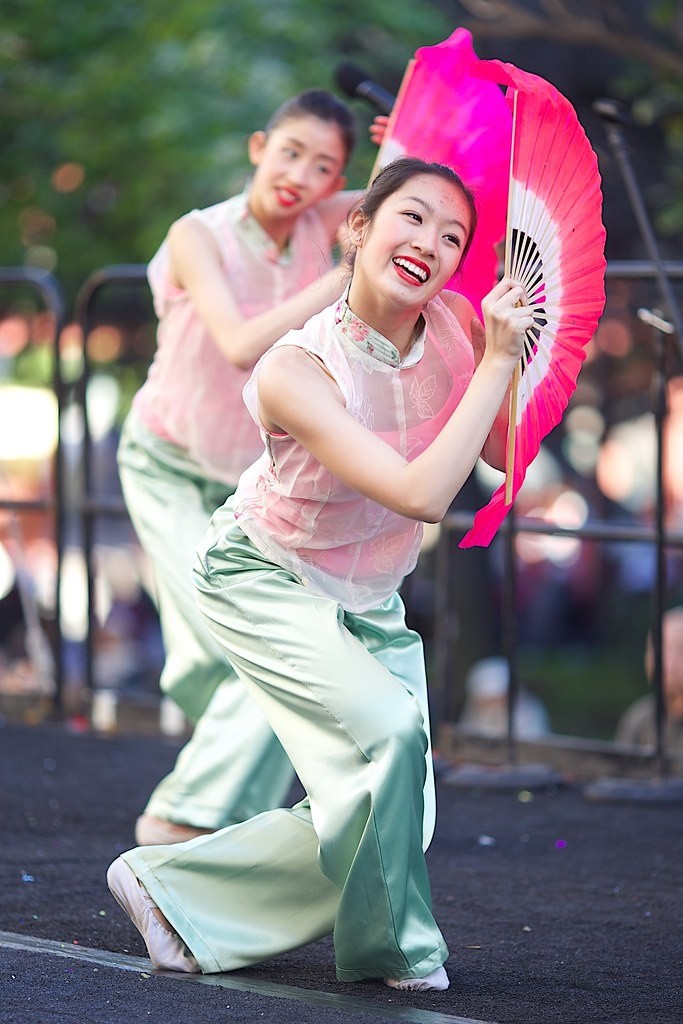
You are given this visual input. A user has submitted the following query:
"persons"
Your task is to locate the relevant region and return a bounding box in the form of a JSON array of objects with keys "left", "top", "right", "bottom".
[
  {"left": 0, "top": 298, "right": 190, "bottom": 744},
  {"left": 113, "top": 93, "right": 396, "bottom": 847},
  {"left": 104, "top": 158, "right": 539, "bottom": 992},
  {"left": 400, "top": 279, "right": 683, "bottom": 727}
]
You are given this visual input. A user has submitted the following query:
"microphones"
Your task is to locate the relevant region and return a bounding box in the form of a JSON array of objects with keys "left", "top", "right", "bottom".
[{"left": 332, "top": 60, "right": 396, "bottom": 117}]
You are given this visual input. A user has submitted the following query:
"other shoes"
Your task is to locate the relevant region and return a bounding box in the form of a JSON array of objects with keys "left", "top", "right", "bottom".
[
  {"left": 382, "top": 965, "right": 451, "bottom": 992},
  {"left": 106, "top": 857, "right": 201, "bottom": 973},
  {"left": 134, "top": 815, "right": 213, "bottom": 846}
]
